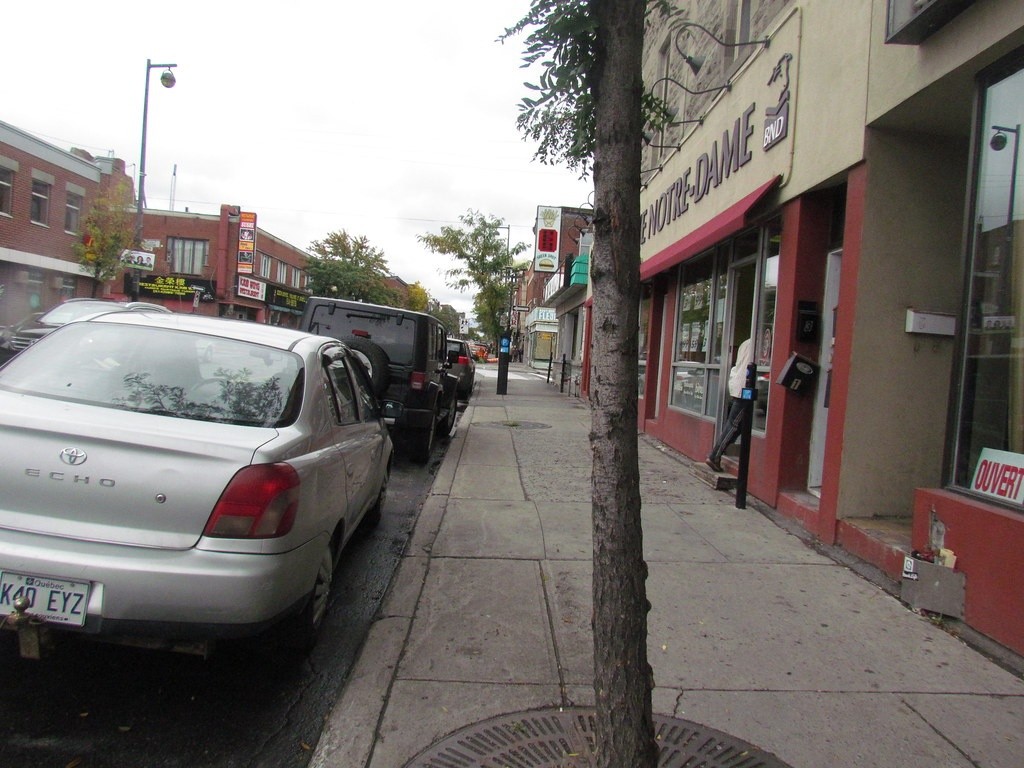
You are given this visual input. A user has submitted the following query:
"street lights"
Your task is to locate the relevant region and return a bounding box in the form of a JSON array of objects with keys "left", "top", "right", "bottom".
[{"left": 131, "top": 59, "right": 177, "bottom": 303}]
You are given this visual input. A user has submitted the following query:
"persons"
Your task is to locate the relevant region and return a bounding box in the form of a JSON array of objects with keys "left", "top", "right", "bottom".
[
  {"left": 706, "top": 337, "right": 751, "bottom": 472},
  {"left": 122, "top": 254, "right": 152, "bottom": 268}
]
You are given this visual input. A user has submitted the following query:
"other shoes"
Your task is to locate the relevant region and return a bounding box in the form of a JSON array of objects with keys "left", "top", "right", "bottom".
[{"left": 706, "top": 453, "right": 724, "bottom": 472}]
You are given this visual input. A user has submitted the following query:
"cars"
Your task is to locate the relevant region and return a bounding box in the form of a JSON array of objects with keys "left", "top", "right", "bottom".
[
  {"left": 442, "top": 339, "right": 475, "bottom": 403},
  {"left": 472, "top": 340, "right": 489, "bottom": 359},
  {"left": 675, "top": 357, "right": 721, "bottom": 403},
  {"left": 0, "top": 314, "right": 404, "bottom": 659}
]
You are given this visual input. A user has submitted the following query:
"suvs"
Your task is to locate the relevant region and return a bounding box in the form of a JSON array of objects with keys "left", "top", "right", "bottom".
[
  {"left": 0, "top": 299, "right": 174, "bottom": 373},
  {"left": 299, "top": 295, "right": 461, "bottom": 462}
]
